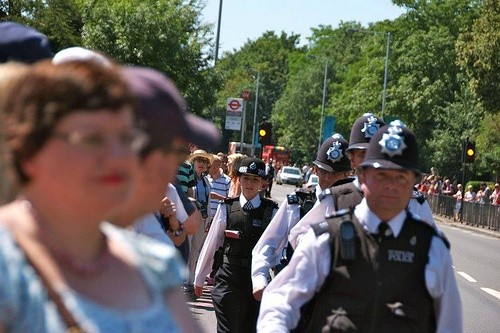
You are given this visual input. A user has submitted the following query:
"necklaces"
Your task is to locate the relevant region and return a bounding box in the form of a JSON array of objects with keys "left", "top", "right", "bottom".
[{"left": 16, "top": 194, "right": 109, "bottom": 275}]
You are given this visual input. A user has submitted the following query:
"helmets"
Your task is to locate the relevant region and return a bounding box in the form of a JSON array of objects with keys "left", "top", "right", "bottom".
[
  {"left": 236, "top": 158, "right": 266, "bottom": 177},
  {"left": 345, "top": 115, "right": 386, "bottom": 152},
  {"left": 313, "top": 133, "right": 353, "bottom": 175},
  {"left": 357, "top": 120, "right": 430, "bottom": 177}
]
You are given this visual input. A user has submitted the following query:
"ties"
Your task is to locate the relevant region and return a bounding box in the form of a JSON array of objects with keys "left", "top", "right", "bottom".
[
  {"left": 243, "top": 201, "right": 254, "bottom": 213},
  {"left": 376, "top": 220, "right": 390, "bottom": 287}
]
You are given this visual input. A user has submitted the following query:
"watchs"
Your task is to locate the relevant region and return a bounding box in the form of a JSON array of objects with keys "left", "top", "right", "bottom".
[{"left": 169, "top": 220, "right": 184, "bottom": 236}]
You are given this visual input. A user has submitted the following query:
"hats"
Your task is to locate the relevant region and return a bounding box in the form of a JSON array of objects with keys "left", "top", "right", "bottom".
[
  {"left": 51, "top": 46, "right": 113, "bottom": 71},
  {"left": 0, "top": 22, "right": 56, "bottom": 64},
  {"left": 117, "top": 65, "right": 221, "bottom": 153},
  {"left": 188, "top": 150, "right": 215, "bottom": 165}
]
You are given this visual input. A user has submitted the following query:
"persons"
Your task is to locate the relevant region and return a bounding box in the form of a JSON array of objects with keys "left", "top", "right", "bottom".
[{"left": 0, "top": 18, "right": 500, "bottom": 333}]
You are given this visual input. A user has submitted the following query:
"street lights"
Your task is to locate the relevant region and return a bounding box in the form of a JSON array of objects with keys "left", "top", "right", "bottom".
[
  {"left": 308, "top": 54, "right": 329, "bottom": 156},
  {"left": 243, "top": 66, "right": 260, "bottom": 158},
  {"left": 346, "top": 28, "right": 390, "bottom": 123}
]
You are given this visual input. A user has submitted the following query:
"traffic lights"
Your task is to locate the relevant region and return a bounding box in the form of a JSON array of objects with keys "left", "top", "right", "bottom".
[
  {"left": 258, "top": 121, "right": 269, "bottom": 144},
  {"left": 465, "top": 143, "right": 475, "bottom": 163}
]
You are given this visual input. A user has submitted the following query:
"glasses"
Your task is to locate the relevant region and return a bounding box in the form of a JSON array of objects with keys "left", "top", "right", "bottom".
[
  {"left": 41, "top": 122, "right": 149, "bottom": 154},
  {"left": 196, "top": 158, "right": 207, "bottom": 163}
]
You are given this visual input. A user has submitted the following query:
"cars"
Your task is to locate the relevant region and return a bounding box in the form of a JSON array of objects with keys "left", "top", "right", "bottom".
[
  {"left": 275, "top": 165, "right": 305, "bottom": 188},
  {"left": 304, "top": 174, "right": 320, "bottom": 190}
]
